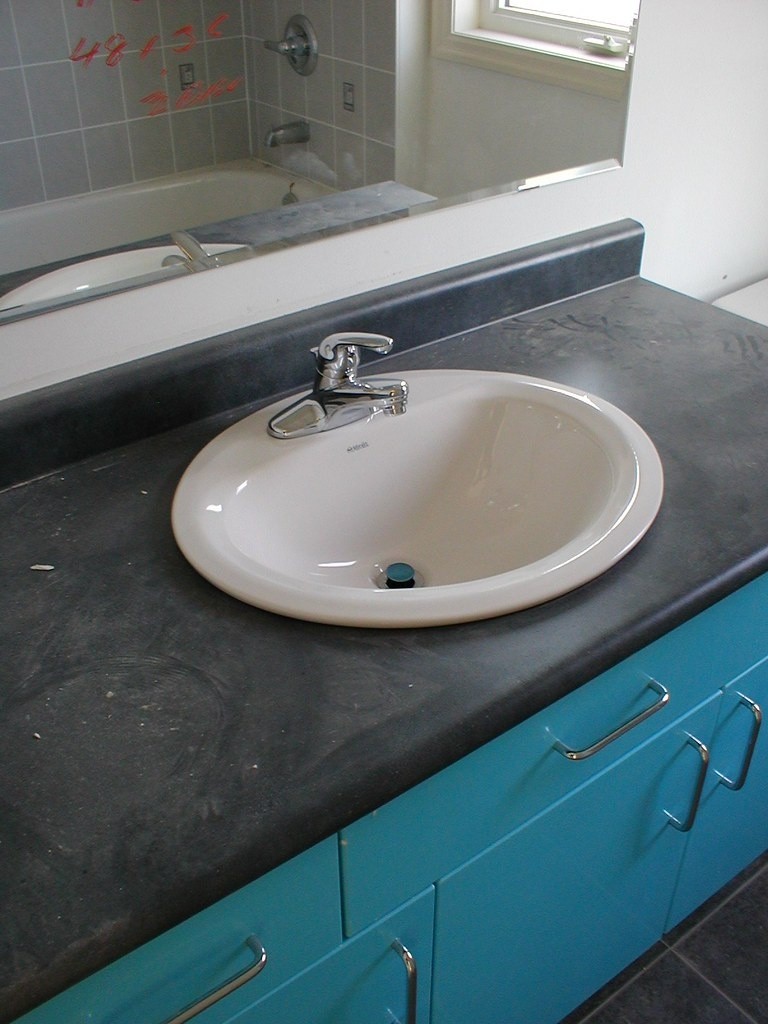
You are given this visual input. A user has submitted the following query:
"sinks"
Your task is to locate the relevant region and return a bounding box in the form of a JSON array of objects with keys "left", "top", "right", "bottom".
[
  {"left": 0, "top": 243, "right": 248, "bottom": 315},
  {"left": 171, "top": 368, "right": 665, "bottom": 629}
]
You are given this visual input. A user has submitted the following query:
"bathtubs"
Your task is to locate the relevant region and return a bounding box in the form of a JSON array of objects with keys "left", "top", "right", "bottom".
[{"left": 0, "top": 157, "right": 345, "bottom": 276}]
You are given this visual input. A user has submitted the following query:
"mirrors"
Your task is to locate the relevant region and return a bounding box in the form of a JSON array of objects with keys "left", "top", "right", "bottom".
[{"left": 0, "top": 0, "right": 640, "bottom": 327}]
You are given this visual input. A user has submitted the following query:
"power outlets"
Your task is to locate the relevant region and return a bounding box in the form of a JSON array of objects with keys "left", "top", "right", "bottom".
[
  {"left": 343, "top": 81, "right": 355, "bottom": 112},
  {"left": 178, "top": 63, "right": 196, "bottom": 90}
]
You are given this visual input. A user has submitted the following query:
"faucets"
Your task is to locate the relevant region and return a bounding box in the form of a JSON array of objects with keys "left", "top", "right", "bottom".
[
  {"left": 160, "top": 229, "right": 209, "bottom": 269},
  {"left": 263, "top": 121, "right": 308, "bottom": 149},
  {"left": 265, "top": 331, "right": 409, "bottom": 441}
]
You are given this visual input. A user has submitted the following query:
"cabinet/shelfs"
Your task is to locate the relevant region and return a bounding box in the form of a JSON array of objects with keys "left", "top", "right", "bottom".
[{"left": 10, "top": 574, "right": 768, "bottom": 1024}]
